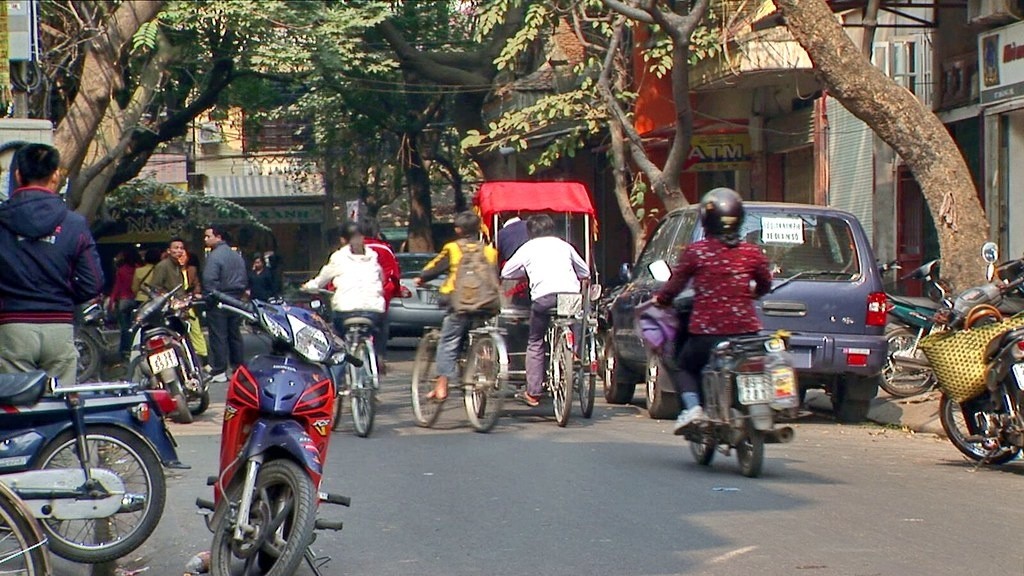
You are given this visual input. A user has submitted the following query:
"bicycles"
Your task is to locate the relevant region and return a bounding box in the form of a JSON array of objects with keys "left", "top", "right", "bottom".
[
  {"left": 298, "top": 287, "right": 381, "bottom": 437},
  {"left": 0, "top": 477, "right": 50, "bottom": 576},
  {"left": 410, "top": 306, "right": 510, "bottom": 434},
  {"left": 540, "top": 307, "right": 577, "bottom": 428}
]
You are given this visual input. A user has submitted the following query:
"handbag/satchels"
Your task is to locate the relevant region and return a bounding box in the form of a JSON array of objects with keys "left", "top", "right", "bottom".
[{"left": 918, "top": 304, "right": 1024, "bottom": 402}]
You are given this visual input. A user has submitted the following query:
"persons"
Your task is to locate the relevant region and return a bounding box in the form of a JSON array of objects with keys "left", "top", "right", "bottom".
[
  {"left": 410, "top": 210, "right": 591, "bottom": 406},
  {"left": 107, "top": 227, "right": 281, "bottom": 394},
  {"left": 297, "top": 216, "right": 401, "bottom": 396},
  {"left": 650, "top": 187, "right": 772, "bottom": 436},
  {"left": 0, "top": 143, "right": 105, "bottom": 533}
]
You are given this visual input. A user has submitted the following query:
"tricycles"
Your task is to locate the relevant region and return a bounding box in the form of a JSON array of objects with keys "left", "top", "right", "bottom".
[{"left": 472, "top": 180, "right": 601, "bottom": 421}]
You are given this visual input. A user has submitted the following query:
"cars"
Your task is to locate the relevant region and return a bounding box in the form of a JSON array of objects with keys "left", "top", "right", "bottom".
[
  {"left": 601, "top": 199, "right": 889, "bottom": 427},
  {"left": 386, "top": 251, "right": 455, "bottom": 340}
]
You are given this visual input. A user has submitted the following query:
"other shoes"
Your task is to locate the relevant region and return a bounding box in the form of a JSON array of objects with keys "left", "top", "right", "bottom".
[
  {"left": 378, "top": 362, "right": 385, "bottom": 373},
  {"left": 209, "top": 370, "right": 227, "bottom": 383},
  {"left": 201, "top": 364, "right": 213, "bottom": 372},
  {"left": 673, "top": 405, "right": 702, "bottom": 435}
]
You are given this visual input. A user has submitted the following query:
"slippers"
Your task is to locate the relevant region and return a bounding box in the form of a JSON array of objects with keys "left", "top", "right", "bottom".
[
  {"left": 514, "top": 392, "right": 539, "bottom": 407},
  {"left": 425, "top": 391, "right": 446, "bottom": 403}
]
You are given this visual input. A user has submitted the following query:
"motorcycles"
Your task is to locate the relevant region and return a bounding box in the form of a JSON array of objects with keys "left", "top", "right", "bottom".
[{"left": 646, "top": 257, "right": 800, "bottom": 479}]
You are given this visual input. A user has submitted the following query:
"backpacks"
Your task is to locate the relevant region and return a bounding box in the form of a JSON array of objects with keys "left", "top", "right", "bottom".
[{"left": 450, "top": 238, "right": 500, "bottom": 314}]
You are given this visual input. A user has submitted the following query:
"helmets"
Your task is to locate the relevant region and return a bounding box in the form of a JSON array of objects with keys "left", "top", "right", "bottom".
[{"left": 700, "top": 188, "right": 745, "bottom": 233}]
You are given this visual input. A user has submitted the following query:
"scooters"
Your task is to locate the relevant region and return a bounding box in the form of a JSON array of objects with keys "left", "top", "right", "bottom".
[
  {"left": 127, "top": 283, "right": 213, "bottom": 424},
  {"left": 0, "top": 370, "right": 193, "bottom": 565},
  {"left": 73, "top": 302, "right": 105, "bottom": 386},
  {"left": 194, "top": 288, "right": 364, "bottom": 576},
  {"left": 876, "top": 255, "right": 1024, "bottom": 398},
  {"left": 939, "top": 241, "right": 1024, "bottom": 466}
]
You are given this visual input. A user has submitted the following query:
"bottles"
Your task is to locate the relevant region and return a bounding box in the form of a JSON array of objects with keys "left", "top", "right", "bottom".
[{"left": 764, "top": 339, "right": 799, "bottom": 410}]
